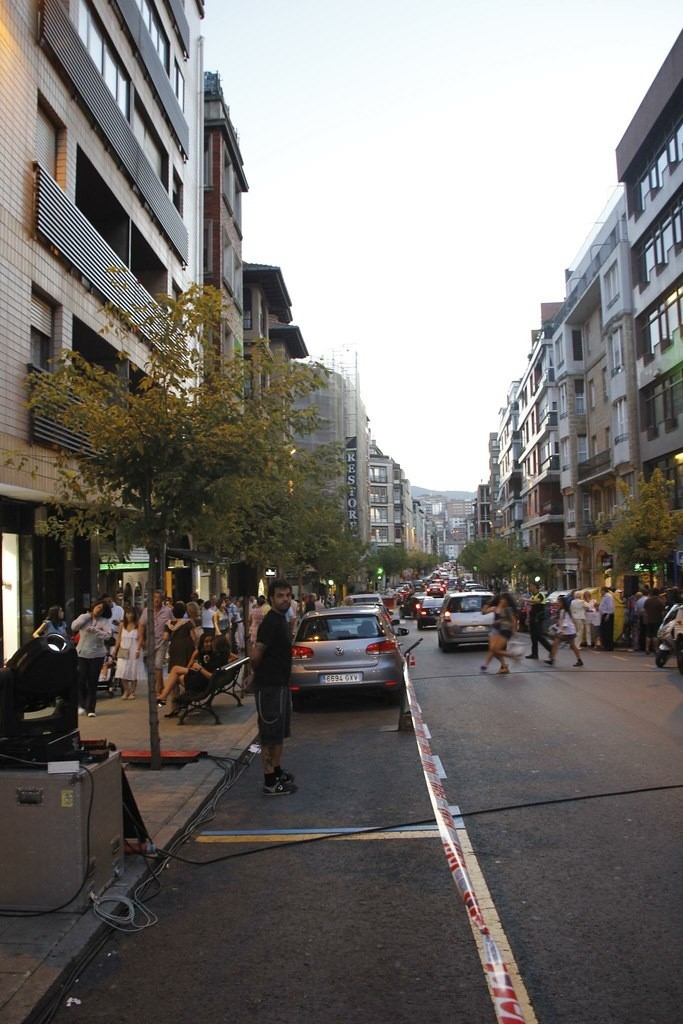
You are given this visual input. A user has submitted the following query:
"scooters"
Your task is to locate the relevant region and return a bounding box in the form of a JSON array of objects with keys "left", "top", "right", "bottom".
[{"left": 654, "top": 602, "right": 681, "bottom": 668}]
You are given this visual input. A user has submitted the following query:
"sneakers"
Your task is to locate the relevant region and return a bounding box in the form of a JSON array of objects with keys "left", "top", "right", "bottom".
[
  {"left": 275, "top": 772, "right": 294, "bottom": 784},
  {"left": 263, "top": 777, "right": 296, "bottom": 795}
]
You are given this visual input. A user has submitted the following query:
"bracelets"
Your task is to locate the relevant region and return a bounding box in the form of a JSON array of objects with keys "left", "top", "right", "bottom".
[{"left": 136, "top": 651, "right": 139, "bottom": 653}]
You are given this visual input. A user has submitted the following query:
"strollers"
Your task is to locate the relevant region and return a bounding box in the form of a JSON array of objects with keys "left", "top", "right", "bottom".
[{"left": 96, "top": 635, "right": 122, "bottom": 699}]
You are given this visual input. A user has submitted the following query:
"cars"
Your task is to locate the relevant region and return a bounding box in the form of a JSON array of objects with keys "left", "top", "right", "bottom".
[
  {"left": 433, "top": 592, "right": 507, "bottom": 654},
  {"left": 544, "top": 589, "right": 576, "bottom": 623},
  {"left": 290, "top": 605, "right": 409, "bottom": 712},
  {"left": 382, "top": 561, "right": 493, "bottom": 605},
  {"left": 343, "top": 603, "right": 399, "bottom": 632},
  {"left": 399, "top": 596, "right": 433, "bottom": 620},
  {"left": 674, "top": 605, "right": 683, "bottom": 677},
  {"left": 417, "top": 597, "right": 446, "bottom": 630},
  {"left": 342, "top": 593, "right": 393, "bottom": 619}
]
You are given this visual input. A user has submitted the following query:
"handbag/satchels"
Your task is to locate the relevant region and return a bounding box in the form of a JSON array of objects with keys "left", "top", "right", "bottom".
[
  {"left": 493, "top": 619, "right": 503, "bottom": 629},
  {"left": 190, "top": 626, "right": 204, "bottom": 647},
  {"left": 547, "top": 623, "right": 560, "bottom": 639},
  {"left": 73, "top": 632, "right": 80, "bottom": 646},
  {"left": 116, "top": 647, "right": 130, "bottom": 659}
]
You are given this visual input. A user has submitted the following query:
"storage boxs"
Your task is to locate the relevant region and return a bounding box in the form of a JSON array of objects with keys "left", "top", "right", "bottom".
[{"left": 0, "top": 751, "right": 123, "bottom": 911}]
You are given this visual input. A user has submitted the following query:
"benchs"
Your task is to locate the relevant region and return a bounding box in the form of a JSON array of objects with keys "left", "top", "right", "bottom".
[{"left": 175, "top": 656, "right": 251, "bottom": 725}]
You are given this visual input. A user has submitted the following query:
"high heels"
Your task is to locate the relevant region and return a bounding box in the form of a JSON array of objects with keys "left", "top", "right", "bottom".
[
  {"left": 157, "top": 699, "right": 166, "bottom": 708},
  {"left": 165, "top": 709, "right": 179, "bottom": 718}
]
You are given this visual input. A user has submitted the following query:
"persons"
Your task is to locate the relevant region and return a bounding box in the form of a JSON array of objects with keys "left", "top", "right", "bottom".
[
  {"left": 139, "top": 588, "right": 174, "bottom": 695},
  {"left": 564, "top": 587, "right": 615, "bottom": 650},
  {"left": 101, "top": 591, "right": 138, "bottom": 700},
  {"left": 544, "top": 597, "right": 584, "bottom": 666},
  {"left": 165, "top": 600, "right": 198, "bottom": 672},
  {"left": 32, "top": 606, "right": 69, "bottom": 639},
  {"left": 164, "top": 593, "right": 354, "bottom": 649},
  {"left": 244, "top": 580, "right": 299, "bottom": 794},
  {"left": 158, "top": 634, "right": 238, "bottom": 717},
  {"left": 616, "top": 586, "right": 683, "bottom": 653},
  {"left": 518, "top": 580, "right": 554, "bottom": 659},
  {"left": 71, "top": 600, "right": 111, "bottom": 716},
  {"left": 480, "top": 591, "right": 521, "bottom": 675}
]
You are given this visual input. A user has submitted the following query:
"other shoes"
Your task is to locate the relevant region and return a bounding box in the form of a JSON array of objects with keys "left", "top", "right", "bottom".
[
  {"left": 573, "top": 661, "right": 583, "bottom": 666},
  {"left": 544, "top": 660, "right": 553, "bottom": 665},
  {"left": 88, "top": 713, "right": 96, "bottom": 717},
  {"left": 496, "top": 666, "right": 509, "bottom": 673},
  {"left": 77, "top": 707, "right": 87, "bottom": 715},
  {"left": 121, "top": 690, "right": 129, "bottom": 699},
  {"left": 128, "top": 692, "right": 135, "bottom": 700},
  {"left": 525, "top": 655, "right": 538, "bottom": 659},
  {"left": 481, "top": 664, "right": 486, "bottom": 669}
]
